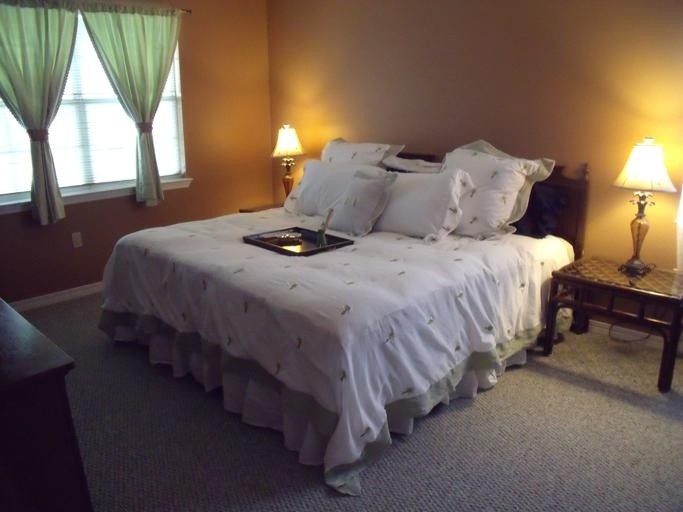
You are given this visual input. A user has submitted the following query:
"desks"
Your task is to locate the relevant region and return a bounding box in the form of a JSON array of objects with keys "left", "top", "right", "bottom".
[
  {"left": 0, "top": 298, "right": 94, "bottom": 512},
  {"left": 238, "top": 201, "right": 283, "bottom": 214},
  {"left": 543, "top": 254, "right": 683, "bottom": 391}
]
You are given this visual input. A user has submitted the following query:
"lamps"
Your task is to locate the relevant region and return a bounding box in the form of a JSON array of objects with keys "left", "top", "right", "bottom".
[
  {"left": 611, "top": 135, "right": 678, "bottom": 275},
  {"left": 269, "top": 121, "right": 305, "bottom": 198}
]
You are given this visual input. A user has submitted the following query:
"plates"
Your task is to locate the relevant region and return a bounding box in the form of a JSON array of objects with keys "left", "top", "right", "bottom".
[{"left": 276, "top": 233, "right": 303, "bottom": 242}]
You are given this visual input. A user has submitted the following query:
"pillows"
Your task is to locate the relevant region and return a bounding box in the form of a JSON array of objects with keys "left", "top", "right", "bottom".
[{"left": 282, "top": 136, "right": 556, "bottom": 246}]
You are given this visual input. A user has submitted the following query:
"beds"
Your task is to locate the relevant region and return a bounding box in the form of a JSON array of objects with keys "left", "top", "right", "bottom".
[{"left": 95, "top": 148, "right": 591, "bottom": 495}]
